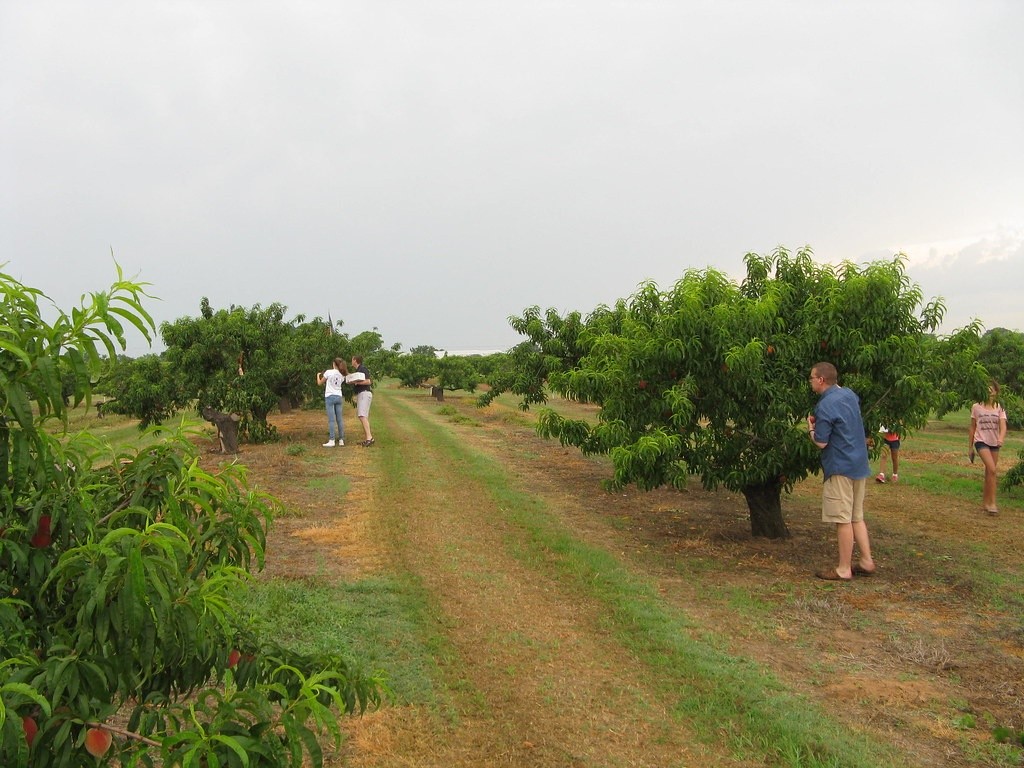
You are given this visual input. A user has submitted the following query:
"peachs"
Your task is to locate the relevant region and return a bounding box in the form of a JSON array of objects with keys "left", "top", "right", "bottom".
[
  {"left": 0, "top": 512, "right": 249, "bottom": 766},
  {"left": 638, "top": 345, "right": 815, "bottom": 429}
]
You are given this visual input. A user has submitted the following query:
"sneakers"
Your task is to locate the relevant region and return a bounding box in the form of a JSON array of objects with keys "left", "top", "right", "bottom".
[
  {"left": 338, "top": 439, "right": 344, "bottom": 445},
  {"left": 322, "top": 440, "right": 335, "bottom": 447},
  {"left": 876, "top": 472, "right": 888, "bottom": 483},
  {"left": 892, "top": 474, "right": 898, "bottom": 482}
]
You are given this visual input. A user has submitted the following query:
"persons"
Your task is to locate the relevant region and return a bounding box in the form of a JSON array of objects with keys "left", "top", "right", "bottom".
[
  {"left": 806, "top": 362, "right": 876, "bottom": 582},
  {"left": 968, "top": 380, "right": 1008, "bottom": 512},
  {"left": 316, "top": 358, "right": 350, "bottom": 447},
  {"left": 867, "top": 415, "right": 900, "bottom": 484},
  {"left": 348, "top": 355, "right": 375, "bottom": 447}
]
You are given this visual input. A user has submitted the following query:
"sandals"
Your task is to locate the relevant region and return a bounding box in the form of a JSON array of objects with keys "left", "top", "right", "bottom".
[
  {"left": 357, "top": 441, "right": 366, "bottom": 445},
  {"left": 364, "top": 438, "right": 375, "bottom": 447}
]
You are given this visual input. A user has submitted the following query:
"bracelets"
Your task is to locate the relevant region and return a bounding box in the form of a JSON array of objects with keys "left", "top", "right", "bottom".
[{"left": 808, "top": 430, "right": 815, "bottom": 435}]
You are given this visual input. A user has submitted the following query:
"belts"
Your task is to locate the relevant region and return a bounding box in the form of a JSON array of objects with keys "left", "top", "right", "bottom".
[{"left": 356, "top": 390, "right": 363, "bottom": 394}]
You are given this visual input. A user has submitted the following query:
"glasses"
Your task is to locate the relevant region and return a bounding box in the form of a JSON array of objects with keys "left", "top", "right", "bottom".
[{"left": 808, "top": 375, "right": 819, "bottom": 379}]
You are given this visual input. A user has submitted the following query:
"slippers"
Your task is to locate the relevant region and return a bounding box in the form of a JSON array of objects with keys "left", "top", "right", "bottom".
[
  {"left": 852, "top": 564, "right": 874, "bottom": 577},
  {"left": 817, "top": 570, "right": 850, "bottom": 580}
]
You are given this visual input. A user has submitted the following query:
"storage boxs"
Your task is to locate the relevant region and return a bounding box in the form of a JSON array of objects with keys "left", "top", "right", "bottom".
[{"left": 346, "top": 373, "right": 365, "bottom": 383}]
